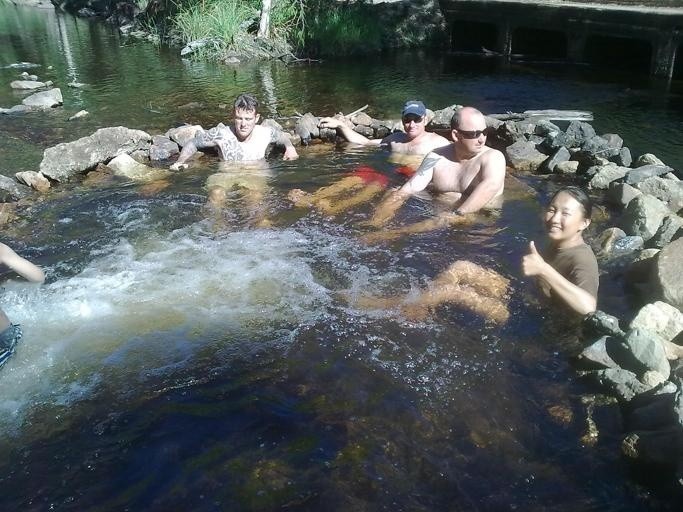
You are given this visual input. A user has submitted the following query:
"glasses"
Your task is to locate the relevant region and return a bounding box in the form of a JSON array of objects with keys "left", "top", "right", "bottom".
[
  {"left": 402, "top": 117, "right": 422, "bottom": 123},
  {"left": 456, "top": 128, "right": 488, "bottom": 139}
]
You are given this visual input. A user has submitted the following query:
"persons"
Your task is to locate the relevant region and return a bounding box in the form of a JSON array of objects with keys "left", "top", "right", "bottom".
[
  {"left": 170, "top": 94, "right": 299, "bottom": 229},
  {"left": 288, "top": 101, "right": 451, "bottom": 219},
  {"left": 358, "top": 106, "right": 506, "bottom": 247},
  {"left": 346, "top": 185, "right": 600, "bottom": 326},
  {"left": 0, "top": 241, "right": 44, "bottom": 370}
]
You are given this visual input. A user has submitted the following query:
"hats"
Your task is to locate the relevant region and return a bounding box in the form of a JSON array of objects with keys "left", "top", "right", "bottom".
[{"left": 402, "top": 100, "right": 426, "bottom": 116}]
